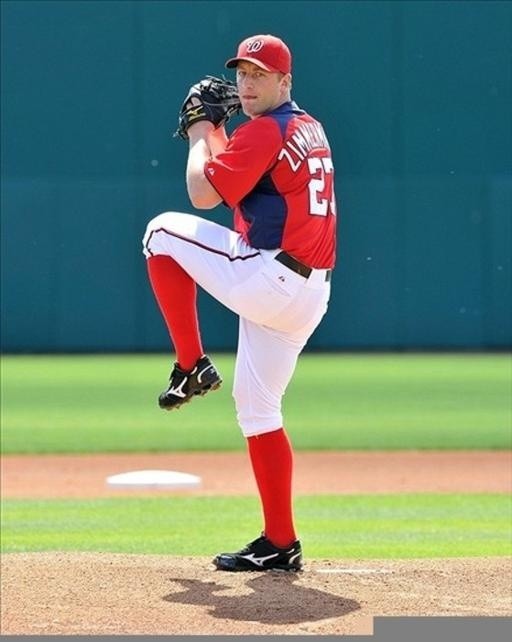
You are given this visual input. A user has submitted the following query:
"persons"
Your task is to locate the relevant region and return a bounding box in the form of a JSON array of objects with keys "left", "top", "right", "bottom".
[{"left": 142, "top": 35, "right": 337, "bottom": 572}]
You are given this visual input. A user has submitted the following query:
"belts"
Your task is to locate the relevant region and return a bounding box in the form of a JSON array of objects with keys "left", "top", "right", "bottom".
[{"left": 275, "top": 252, "right": 331, "bottom": 284}]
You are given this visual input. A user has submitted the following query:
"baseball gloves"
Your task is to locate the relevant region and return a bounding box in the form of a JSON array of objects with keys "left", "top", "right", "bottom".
[{"left": 176, "top": 74, "right": 241, "bottom": 140}]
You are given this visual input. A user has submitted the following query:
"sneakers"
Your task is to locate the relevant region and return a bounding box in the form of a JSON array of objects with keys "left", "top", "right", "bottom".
[
  {"left": 159, "top": 355, "right": 223, "bottom": 411},
  {"left": 216, "top": 536, "right": 301, "bottom": 572}
]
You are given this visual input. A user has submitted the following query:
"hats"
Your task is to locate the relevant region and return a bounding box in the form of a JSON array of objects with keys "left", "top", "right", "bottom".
[{"left": 225, "top": 34, "right": 291, "bottom": 74}]
